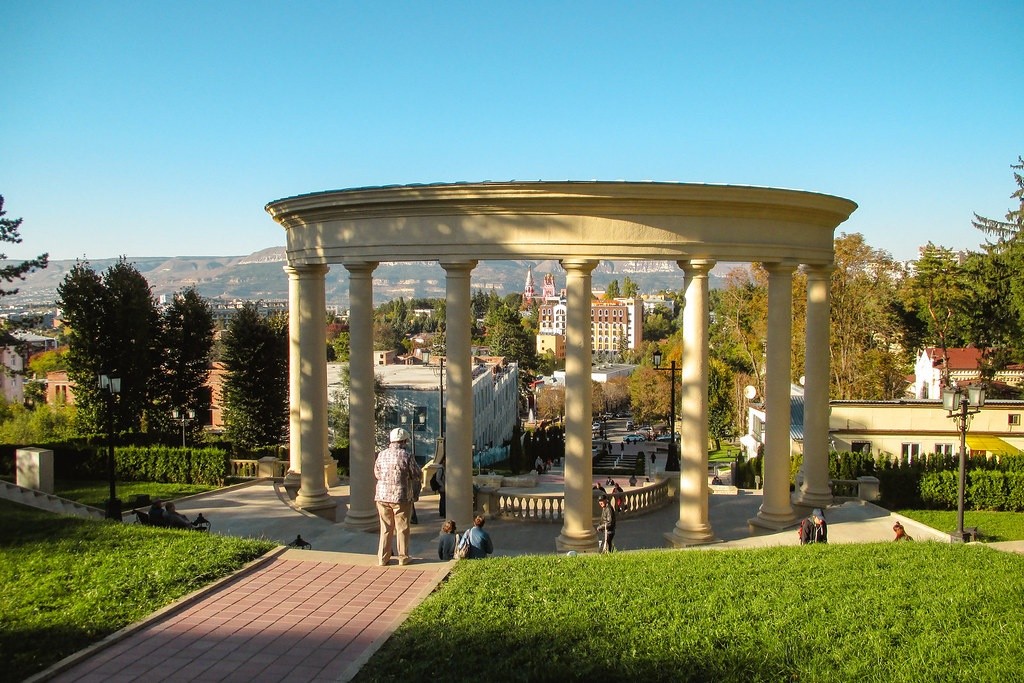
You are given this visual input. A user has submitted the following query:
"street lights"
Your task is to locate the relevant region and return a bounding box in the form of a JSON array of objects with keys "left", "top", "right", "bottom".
[
  {"left": 940, "top": 384, "right": 987, "bottom": 547},
  {"left": 651, "top": 350, "right": 682, "bottom": 472},
  {"left": 422, "top": 347, "right": 447, "bottom": 463},
  {"left": 96, "top": 369, "right": 124, "bottom": 523},
  {"left": 170, "top": 408, "right": 196, "bottom": 448}
]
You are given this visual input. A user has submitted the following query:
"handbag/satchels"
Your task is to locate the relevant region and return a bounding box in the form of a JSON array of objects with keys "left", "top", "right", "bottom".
[
  {"left": 454, "top": 534, "right": 461, "bottom": 560},
  {"left": 460, "top": 529, "right": 470, "bottom": 557},
  {"left": 407, "top": 454, "right": 421, "bottom": 502}
]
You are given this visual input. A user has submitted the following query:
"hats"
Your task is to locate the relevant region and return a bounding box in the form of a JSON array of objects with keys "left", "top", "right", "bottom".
[
  {"left": 813, "top": 509, "right": 824, "bottom": 519},
  {"left": 389, "top": 428, "right": 410, "bottom": 443},
  {"left": 599, "top": 495, "right": 607, "bottom": 501},
  {"left": 152, "top": 496, "right": 163, "bottom": 503}
]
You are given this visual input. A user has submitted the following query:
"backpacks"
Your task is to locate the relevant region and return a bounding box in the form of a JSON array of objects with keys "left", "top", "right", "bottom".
[
  {"left": 798, "top": 520, "right": 806, "bottom": 544},
  {"left": 430, "top": 468, "right": 443, "bottom": 491}
]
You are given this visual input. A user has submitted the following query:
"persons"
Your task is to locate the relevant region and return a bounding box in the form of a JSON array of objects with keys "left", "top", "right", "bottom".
[
  {"left": 535, "top": 455, "right": 552, "bottom": 475},
  {"left": 597, "top": 495, "right": 617, "bottom": 553},
  {"left": 608, "top": 441, "right": 613, "bottom": 454},
  {"left": 625, "top": 438, "right": 631, "bottom": 445},
  {"left": 374, "top": 428, "right": 423, "bottom": 567},
  {"left": 801, "top": 509, "right": 828, "bottom": 545},
  {"left": 621, "top": 442, "right": 624, "bottom": 451},
  {"left": 592, "top": 475, "right": 650, "bottom": 511},
  {"left": 488, "top": 468, "right": 496, "bottom": 475},
  {"left": 633, "top": 438, "right": 637, "bottom": 444},
  {"left": 712, "top": 476, "right": 723, "bottom": 485},
  {"left": 651, "top": 453, "right": 656, "bottom": 464},
  {"left": 435, "top": 459, "right": 445, "bottom": 515},
  {"left": 164, "top": 501, "right": 193, "bottom": 529},
  {"left": 149, "top": 499, "right": 165, "bottom": 514},
  {"left": 438, "top": 520, "right": 457, "bottom": 560},
  {"left": 602, "top": 442, "right": 607, "bottom": 450},
  {"left": 892, "top": 522, "right": 914, "bottom": 541},
  {"left": 459, "top": 517, "right": 493, "bottom": 559}
]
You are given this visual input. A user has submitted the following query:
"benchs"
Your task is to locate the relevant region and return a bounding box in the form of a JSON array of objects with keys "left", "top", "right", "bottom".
[{"left": 133, "top": 509, "right": 153, "bottom": 526}]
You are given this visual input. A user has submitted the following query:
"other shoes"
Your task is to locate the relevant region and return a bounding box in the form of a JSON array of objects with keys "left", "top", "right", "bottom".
[
  {"left": 440, "top": 513, "right": 444, "bottom": 516},
  {"left": 410, "top": 521, "right": 417, "bottom": 524},
  {"left": 379, "top": 555, "right": 390, "bottom": 566},
  {"left": 399, "top": 556, "right": 412, "bottom": 565}
]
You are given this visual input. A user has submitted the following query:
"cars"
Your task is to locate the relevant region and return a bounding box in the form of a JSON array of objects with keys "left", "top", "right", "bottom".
[{"left": 591, "top": 411, "right": 680, "bottom": 444}]
